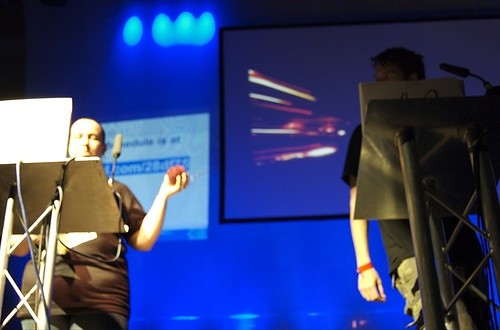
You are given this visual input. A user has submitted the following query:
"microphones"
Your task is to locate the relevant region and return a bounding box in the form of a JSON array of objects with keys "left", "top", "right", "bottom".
[
  {"left": 108, "top": 134, "right": 123, "bottom": 184},
  {"left": 439, "top": 62, "right": 491, "bottom": 90}
]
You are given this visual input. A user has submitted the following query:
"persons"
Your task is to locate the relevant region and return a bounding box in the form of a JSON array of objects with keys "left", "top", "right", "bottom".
[
  {"left": 8, "top": 117, "right": 191, "bottom": 330},
  {"left": 339, "top": 44, "right": 496, "bottom": 329}
]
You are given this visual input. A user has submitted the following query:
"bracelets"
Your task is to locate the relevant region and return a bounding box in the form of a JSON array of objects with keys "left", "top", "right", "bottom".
[{"left": 356, "top": 263, "right": 374, "bottom": 274}]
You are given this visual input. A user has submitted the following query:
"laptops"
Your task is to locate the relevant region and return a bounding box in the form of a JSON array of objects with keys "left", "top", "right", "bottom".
[
  {"left": 359, "top": 77, "right": 465, "bottom": 126},
  {"left": 0, "top": 98, "right": 73, "bottom": 163}
]
